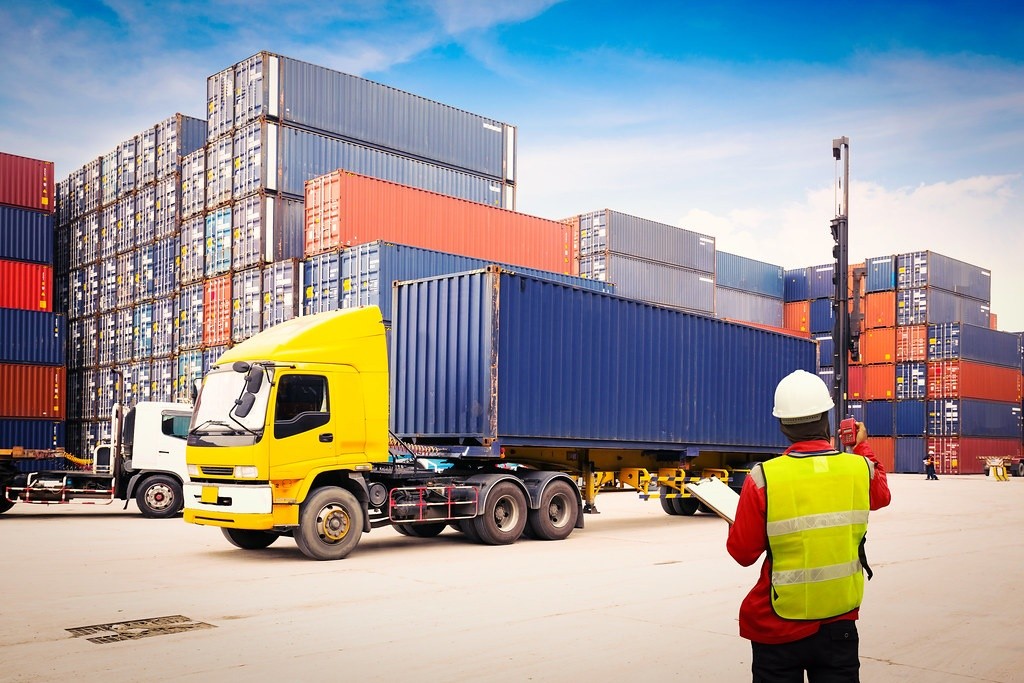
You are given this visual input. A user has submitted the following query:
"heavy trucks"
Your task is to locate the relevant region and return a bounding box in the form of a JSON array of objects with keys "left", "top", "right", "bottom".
[
  {"left": 975, "top": 455, "right": 1024, "bottom": 477},
  {"left": 0, "top": 401, "right": 196, "bottom": 519},
  {"left": 181, "top": 264, "right": 821, "bottom": 561}
]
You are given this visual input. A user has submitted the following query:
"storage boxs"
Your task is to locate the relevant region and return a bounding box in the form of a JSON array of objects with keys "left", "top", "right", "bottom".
[{"left": 0, "top": 49, "right": 1024, "bottom": 476}]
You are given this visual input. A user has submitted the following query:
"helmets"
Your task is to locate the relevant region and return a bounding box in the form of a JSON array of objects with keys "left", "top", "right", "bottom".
[{"left": 771, "top": 369, "right": 835, "bottom": 425}]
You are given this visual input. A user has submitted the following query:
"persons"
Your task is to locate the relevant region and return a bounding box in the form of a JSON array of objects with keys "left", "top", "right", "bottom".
[
  {"left": 921, "top": 449, "right": 939, "bottom": 480},
  {"left": 726, "top": 370, "right": 892, "bottom": 683}
]
